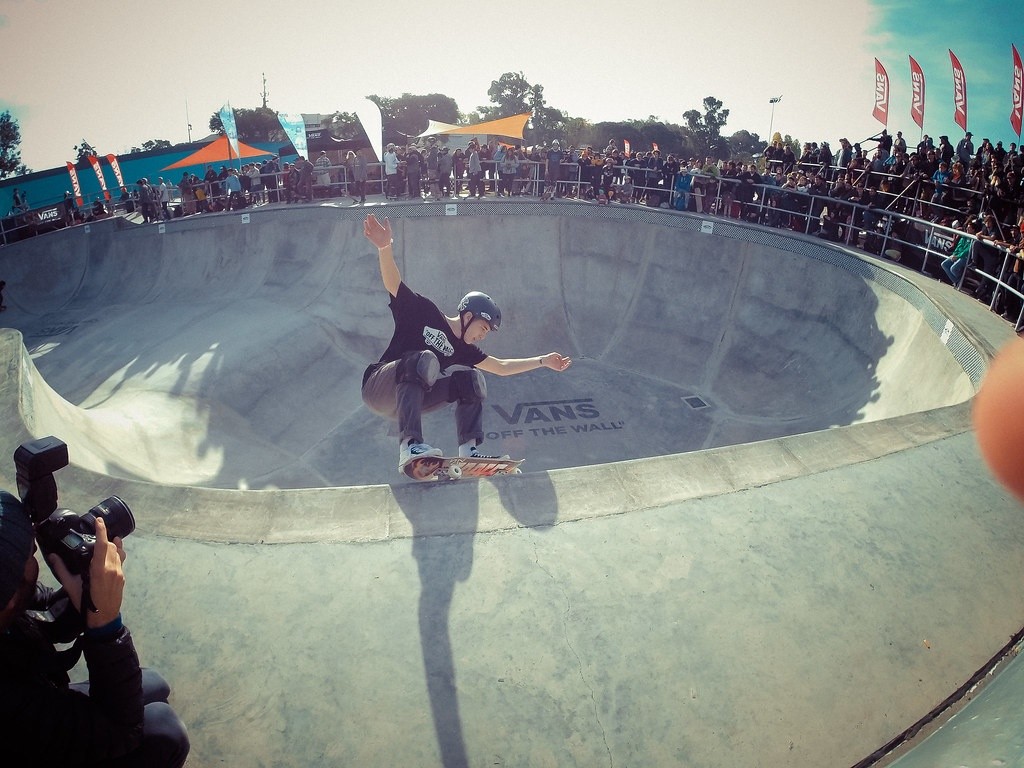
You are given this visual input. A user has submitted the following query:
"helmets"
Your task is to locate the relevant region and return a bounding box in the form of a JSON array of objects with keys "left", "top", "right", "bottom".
[{"left": 457, "top": 291, "right": 501, "bottom": 332}]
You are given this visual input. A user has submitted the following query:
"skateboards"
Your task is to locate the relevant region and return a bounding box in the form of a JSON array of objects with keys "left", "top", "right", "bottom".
[{"left": 402, "top": 454, "right": 526, "bottom": 481}]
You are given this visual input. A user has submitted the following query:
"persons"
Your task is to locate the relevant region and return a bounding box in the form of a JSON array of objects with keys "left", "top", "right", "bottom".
[
  {"left": 343, "top": 149, "right": 367, "bottom": 204},
  {"left": 360, "top": 213, "right": 572, "bottom": 475},
  {"left": 0, "top": 489, "right": 191, "bottom": 767},
  {"left": 179, "top": 150, "right": 331, "bottom": 216},
  {"left": 383, "top": 130, "right": 1024, "bottom": 322},
  {"left": 12, "top": 187, "right": 31, "bottom": 212},
  {"left": 65, "top": 177, "right": 172, "bottom": 223}
]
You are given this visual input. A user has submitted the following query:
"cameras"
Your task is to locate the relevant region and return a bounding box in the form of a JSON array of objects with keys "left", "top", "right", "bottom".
[{"left": 13, "top": 435, "right": 136, "bottom": 586}]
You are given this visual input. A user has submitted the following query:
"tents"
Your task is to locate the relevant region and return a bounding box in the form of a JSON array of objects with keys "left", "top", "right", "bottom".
[
  {"left": 279, "top": 127, "right": 416, "bottom": 200},
  {"left": 162, "top": 134, "right": 269, "bottom": 169},
  {"left": 417, "top": 112, "right": 534, "bottom": 140}
]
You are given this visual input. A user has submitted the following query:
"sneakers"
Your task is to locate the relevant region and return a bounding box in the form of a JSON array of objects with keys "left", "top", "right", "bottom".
[
  {"left": 469, "top": 445, "right": 510, "bottom": 461},
  {"left": 398, "top": 439, "right": 442, "bottom": 474}
]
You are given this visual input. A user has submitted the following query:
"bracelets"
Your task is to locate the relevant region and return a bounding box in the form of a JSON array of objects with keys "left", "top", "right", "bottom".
[
  {"left": 378, "top": 238, "right": 393, "bottom": 250},
  {"left": 539, "top": 355, "right": 543, "bottom": 364}
]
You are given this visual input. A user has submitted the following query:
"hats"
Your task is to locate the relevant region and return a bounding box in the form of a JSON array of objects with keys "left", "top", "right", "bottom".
[
  {"left": 966, "top": 132, "right": 973, "bottom": 137},
  {"left": 0, "top": 491, "right": 32, "bottom": 614}
]
